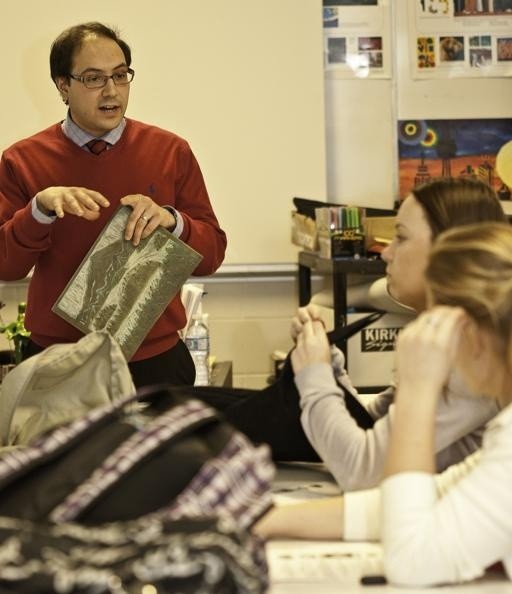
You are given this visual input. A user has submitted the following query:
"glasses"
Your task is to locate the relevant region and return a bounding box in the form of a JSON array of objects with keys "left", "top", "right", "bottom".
[{"left": 70, "top": 68, "right": 134, "bottom": 88}]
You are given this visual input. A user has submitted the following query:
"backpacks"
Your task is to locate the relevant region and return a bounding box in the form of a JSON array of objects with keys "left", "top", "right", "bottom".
[{"left": 0, "top": 381, "right": 280, "bottom": 594}]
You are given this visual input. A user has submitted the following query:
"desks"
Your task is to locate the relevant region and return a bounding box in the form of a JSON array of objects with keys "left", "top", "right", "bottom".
[{"left": 295, "top": 252, "right": 386, "bottom": 372}]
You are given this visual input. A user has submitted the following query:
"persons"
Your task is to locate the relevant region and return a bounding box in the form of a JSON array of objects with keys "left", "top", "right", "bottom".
[
  {"left": 0, "top": 21, "right": 229, "bottom": 392},
  {"left": 288, "top": 174, "right": 508, "bottom": 492},
  {"left": 250, "top": 222, "right": 511, "bottom": 588}
]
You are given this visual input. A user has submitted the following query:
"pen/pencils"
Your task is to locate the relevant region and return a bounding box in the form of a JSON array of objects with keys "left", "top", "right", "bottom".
[{"left": 331, "top": 207, "right": 366, "bottom": 230}]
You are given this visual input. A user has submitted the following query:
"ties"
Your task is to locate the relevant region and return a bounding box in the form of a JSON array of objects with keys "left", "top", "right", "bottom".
[{"left": 85, "top": 139, "right": 108, "bottom": 156}]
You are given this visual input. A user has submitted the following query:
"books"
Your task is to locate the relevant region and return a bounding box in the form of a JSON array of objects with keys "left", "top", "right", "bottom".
[
  {"left": 313, "top": 203, "right": 367, "bottom": 236},
  {"left": 50, "top": 199, "right": 205, "bottom": 369}
]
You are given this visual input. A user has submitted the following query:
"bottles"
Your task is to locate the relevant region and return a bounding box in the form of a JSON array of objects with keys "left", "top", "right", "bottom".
[
  {"left": 185, "top": 313, "right": 209, "bottom": 386},
  {"left": 13, "top": 301, "right": 26, "bottom": 363}
]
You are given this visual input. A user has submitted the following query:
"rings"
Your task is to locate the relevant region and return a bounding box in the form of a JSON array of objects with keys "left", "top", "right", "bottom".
[
  {"left": 426, "top": 313, "right": 442, "bottom": 325},
  {"left": 139, "top": 214, "right": 148, "bottom": 221}
]
